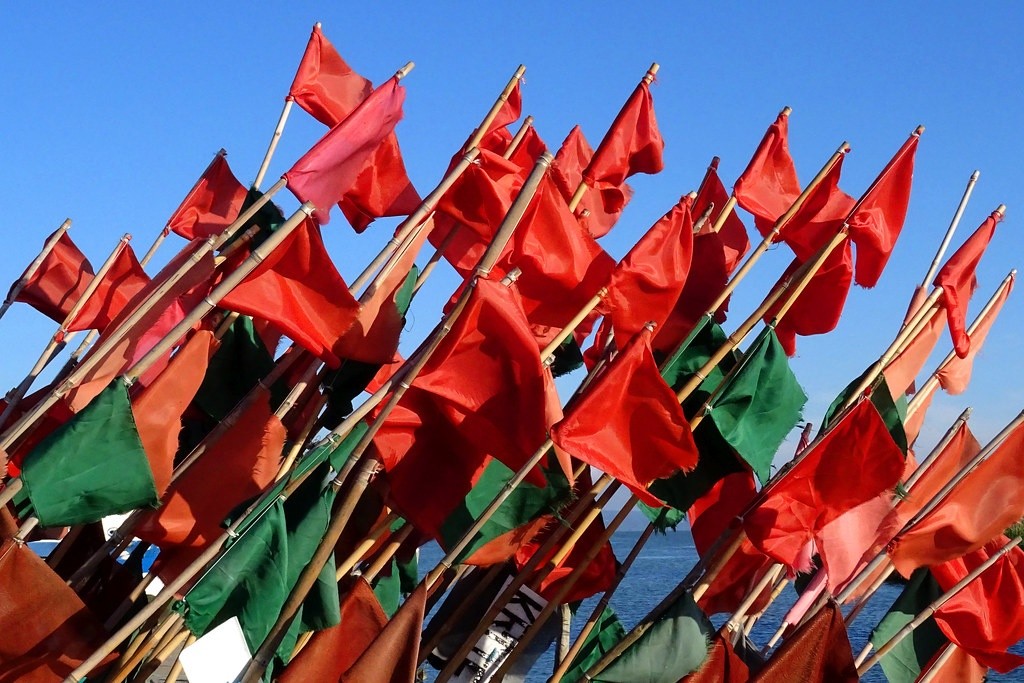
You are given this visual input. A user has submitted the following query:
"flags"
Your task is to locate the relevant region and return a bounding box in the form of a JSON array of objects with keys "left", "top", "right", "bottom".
[
  {"left": 336, "top": 129, "right": 423, "bottom": 234},
  {"left": 584, "top": 80, "right": 666, "bottom": 184},
  {"left": 782, "top": 157, "right": 857, "bottom": 276},
  {"left": 734, "top": 113, "right": 801, "bottom": 242},
  {"left": 554, "top": 129, "right": 633, "bottom": 239},
  {"left": 0, "top": 88, "right": 1024, "bottom": 683},
  {"left": 286, "top": 25, "right": 375, "bottom": 128},
  {"left": 283, "top": 77, "right": 405, "bottom": 222},
  {"left": 850, "top": 136, "right": 917, "bottom": 287}
]
